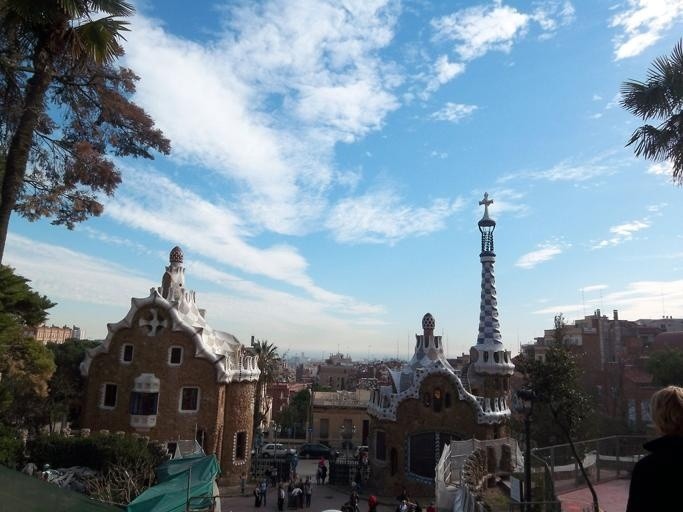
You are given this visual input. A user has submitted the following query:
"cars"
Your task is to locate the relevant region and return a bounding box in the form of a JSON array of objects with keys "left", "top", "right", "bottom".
[
  {"left": 262, "top": 444, "right": 295, "bottom": 458},
  {"left": 353, "top": 446, "right": 368, "bottom": 458},
  {"left": 299, "top": 444, "right": 340, "bottom": 459}
]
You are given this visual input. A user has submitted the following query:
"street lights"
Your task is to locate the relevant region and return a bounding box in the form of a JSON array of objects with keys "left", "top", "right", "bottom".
[
  {"left": 339, "top": 425, "right": 355, "bottom": 463},
  {"left": 516, "top": 388, "right": 535, "bottom": 512}
]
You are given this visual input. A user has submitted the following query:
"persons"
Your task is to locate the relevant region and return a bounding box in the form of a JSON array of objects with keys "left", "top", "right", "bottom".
[
  {"left": 154, "top": 444, "right": 164, "bottom": 456},
  {"left": 240, "top": 453, "right": 436, "bottom": 511},
  {"left": 626, "top": 387, "right": 683, "bottom": 512},
  {"left": 22, "top": 460, "right": 37, "bottom": 477}
]
